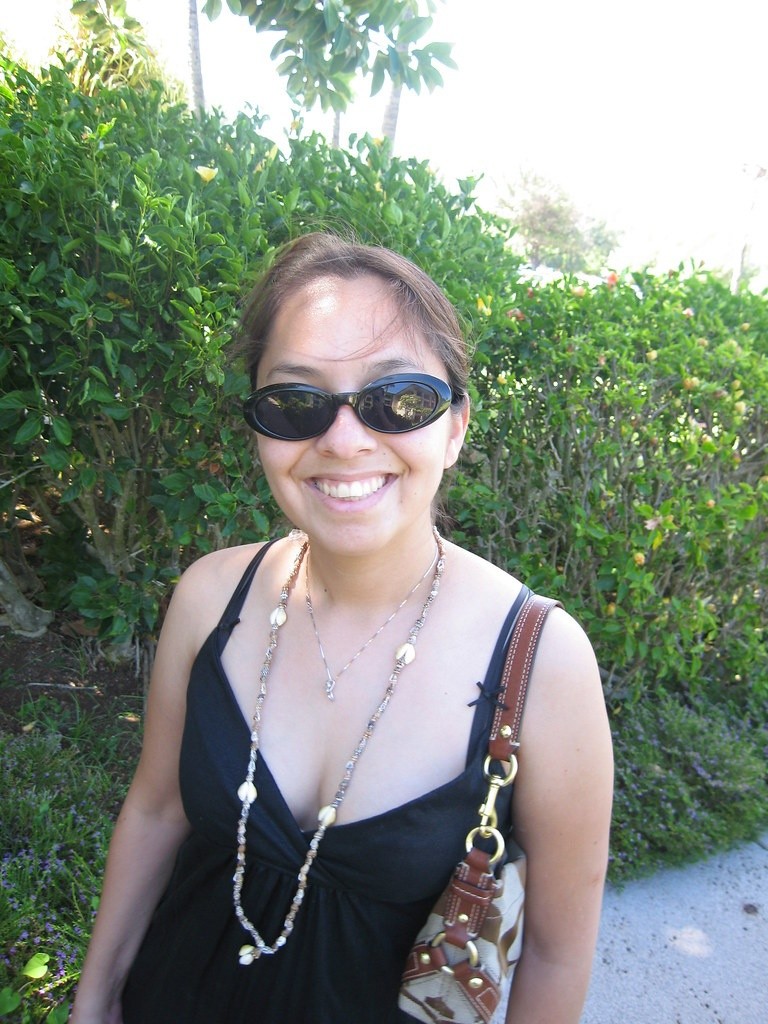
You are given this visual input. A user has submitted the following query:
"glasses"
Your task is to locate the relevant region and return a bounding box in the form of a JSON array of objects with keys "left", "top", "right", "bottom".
[{"left": 241, "top": 372, "right": 453, "bottom": 441}]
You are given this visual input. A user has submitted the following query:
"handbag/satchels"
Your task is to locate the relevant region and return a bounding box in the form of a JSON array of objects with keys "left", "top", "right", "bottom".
[{"left": 401, "top": 595, "right": 566, "bottom": 1024}]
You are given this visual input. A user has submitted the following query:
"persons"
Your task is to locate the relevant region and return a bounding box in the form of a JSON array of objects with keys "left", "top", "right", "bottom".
[{"left": 71, "top": 232, "right": 616, "bottom": 1024}]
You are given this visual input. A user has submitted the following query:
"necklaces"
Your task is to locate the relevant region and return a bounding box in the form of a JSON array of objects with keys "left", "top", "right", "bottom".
[
  {"left": 304, "top": 543, "right": 439, "bottom": 702},
  {"left": 233, "top": 526, "right": 447, "bottom": 966}
]
